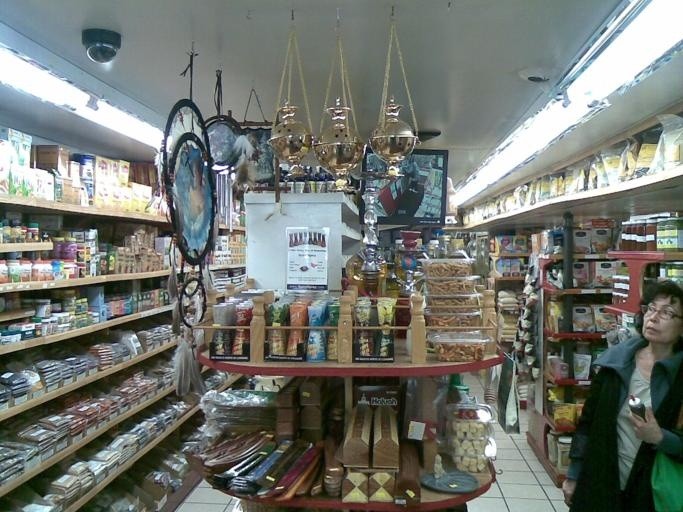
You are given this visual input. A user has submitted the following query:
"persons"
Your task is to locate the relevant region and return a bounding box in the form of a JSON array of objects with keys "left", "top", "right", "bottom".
[{"left": 561, "top": 278, "right": 683, "bottom": 512}]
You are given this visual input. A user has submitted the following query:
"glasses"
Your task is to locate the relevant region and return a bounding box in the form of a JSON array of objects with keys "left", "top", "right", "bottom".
[{"left": 641, "top": 305, "right": 682, "bottom": 320}]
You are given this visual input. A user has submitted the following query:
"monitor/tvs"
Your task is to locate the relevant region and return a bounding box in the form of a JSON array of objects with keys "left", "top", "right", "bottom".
[{"left": 359, "top": 147, "right": 448, "bottom": 226}]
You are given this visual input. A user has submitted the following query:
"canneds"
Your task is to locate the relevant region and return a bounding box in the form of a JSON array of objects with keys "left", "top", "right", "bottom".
[
  {"left": 612, "top": 275, "right": 630, "bottom": 305},
  {"left": 0, "top": 236, "right": 100, "bottom": 336},
  {"left": 622, "top": 217, "right": 683, "bottom": 252},
  {"left": 659, "top": 260, "right": 683, "bottom": 278}
]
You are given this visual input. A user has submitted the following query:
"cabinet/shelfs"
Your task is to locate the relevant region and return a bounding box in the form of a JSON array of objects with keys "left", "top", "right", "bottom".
[{"left": 0, "top": 45, "right": 683, "bottom": 511}]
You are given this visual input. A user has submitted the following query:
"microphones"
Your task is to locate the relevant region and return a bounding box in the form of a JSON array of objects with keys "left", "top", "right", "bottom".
[{"left": 82, "top": 29, "right": 121, "bottom": 64}]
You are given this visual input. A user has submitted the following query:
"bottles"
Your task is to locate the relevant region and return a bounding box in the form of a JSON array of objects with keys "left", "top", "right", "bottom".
[
  {"left": 276, "top": 165, "right": 335, "bottom": 193},
  {"left": 387, "top": 262, "right": 398, "bottom": 290}
]
